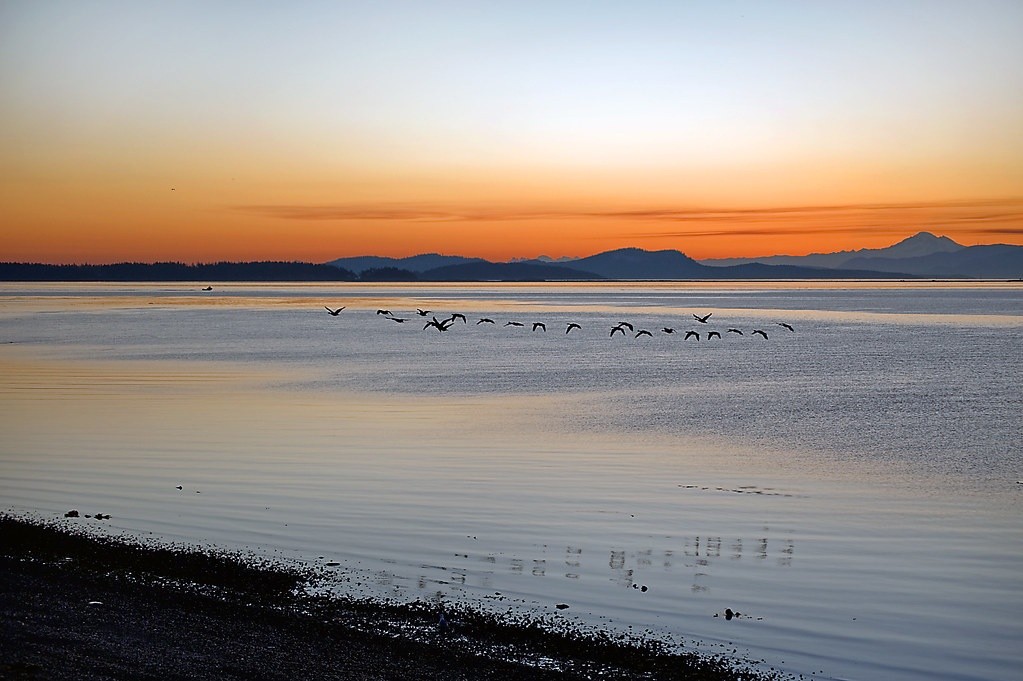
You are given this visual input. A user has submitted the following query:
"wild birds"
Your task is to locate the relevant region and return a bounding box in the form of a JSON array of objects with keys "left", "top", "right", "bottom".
[
  {"left": 708, "top": 331, "right": 722, "bottom": 340},
  {"left": 684, "top": 330, "right": 700, "bottom": 341},
  {"left": 385, "top": 317, "right": 412, "bottom": 322},
  {"left": 477, "top": 319, "right": 495, "bottom": 324},
  {"left": 610, "top": 321, "right": 634, "bottom": 336},
  {"left": 532, "top": 322, "right": 547, "bottom": 332},
  {"left": 324, "top": 306, "right": 346, "bottom": 316},
  {"left": 503, "top": 322, "right": 525, "bottom": 326},
  {"left": 777, "top": 322, "right": 794, "bottom": 331},
  {"left": 661, "top": 327, "right": 677, "bottom": 333},
  {"left": 452, "top": 313, "right": 466, "bottom": 324},
  {"left": 423, "top": 316, "right": 454, "bottom": 332},
  {"left": 416, "top": 308, "right": 431, "bottom": 316},
  {"left": 565, "top": 323, "right": 581, "bottom": 334},
  {"left": 635, "top": 330, "right": 653, "bottom": 338},
  {"left": 377, "top": 310, "right": 394, "bottom": 316},
  {"left": 693, "top": 313, "right": 712, "bottom": 323},
  {"left": 752, "top": 330, "right": 768, "bottom": 340},
  {"left": 727, "top": 328, "right": 743, "bottom": 335}
]
